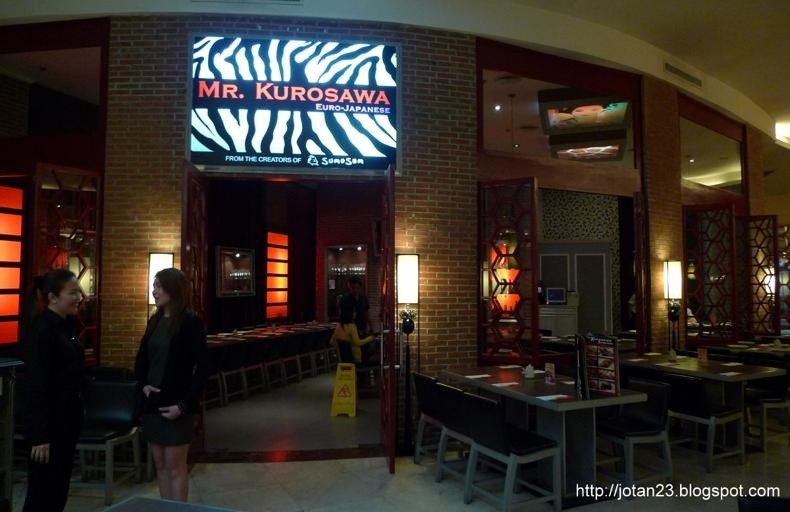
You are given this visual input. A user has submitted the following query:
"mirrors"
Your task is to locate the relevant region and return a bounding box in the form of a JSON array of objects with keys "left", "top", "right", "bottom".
[{"left": 215, "top": 244, "right": 257, "bottom": 298}]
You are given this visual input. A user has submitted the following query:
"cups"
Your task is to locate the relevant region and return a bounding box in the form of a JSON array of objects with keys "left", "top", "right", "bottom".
[{"left": 668, "top": 349, "right": 677, "bottom": 362}]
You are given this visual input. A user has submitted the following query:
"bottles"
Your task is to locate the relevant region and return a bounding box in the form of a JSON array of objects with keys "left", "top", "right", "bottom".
[{"left": 521, "top": 363, "right": 535, "bottom": 379}]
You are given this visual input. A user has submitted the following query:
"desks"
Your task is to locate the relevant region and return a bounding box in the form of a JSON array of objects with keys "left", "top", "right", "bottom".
[
  {"left": 441, "top": 336, "right": 790, "bottom": 512},
  {"left": 102, "top": 495, "right": 241, "bottom": 512},
  {"left": 203, "top": 320, "right": 342, "bottom": 394}
]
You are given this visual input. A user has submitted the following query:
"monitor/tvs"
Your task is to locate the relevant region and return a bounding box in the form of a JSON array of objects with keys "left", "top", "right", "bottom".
[
  {"left": 552, "top": 141, "right": 626, "bottom": 164},
  {"left": 186, "top": 32, "right": 405, "bottom": 177},
  {"left": 538, "top": 89, "right": 633, "bottom": 136},
  {"left": 547, "top": 288, "right": 566, "bottom": 303}
]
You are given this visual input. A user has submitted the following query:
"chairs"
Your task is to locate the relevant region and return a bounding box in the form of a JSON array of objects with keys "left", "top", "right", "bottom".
[
  {"left": 0, "top": 364, "right": 151, "bottom": 506},
  {"left": 410, "top": 369, "right": 563, "bottom": 512},
  {"left": 441, "top": 336, "right": 790, "bottom": 512},
  {"left": 334, "top": 338, "right": 380, "bottom": 397},
  {"left": 189, "top": 328, "right": 342, "bottom": 411}
]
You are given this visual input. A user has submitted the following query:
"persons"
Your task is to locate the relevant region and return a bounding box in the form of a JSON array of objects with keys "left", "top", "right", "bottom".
[
  {"left": 129, "top": 266, "right": 204, "bottom": 503},
  {"left": 329, "top": 301, "right": 377, "bottom": 369},
  {"left": 337, "top": 275, "right": 374, "bottom": 333},
  {"left": 18, "top": 268, "right": 82, "bottom": 511}
]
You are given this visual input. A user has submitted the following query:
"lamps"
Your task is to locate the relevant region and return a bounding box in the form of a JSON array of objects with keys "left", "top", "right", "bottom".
[
  {"left": 395, "top": 254, "right": 420, "bottom": 457},
  {"left": 147, "top": 252, "right": 175, "bottom": 334},
  {"left": 662, "top": 259, "right": 682, "bottom": 354}
]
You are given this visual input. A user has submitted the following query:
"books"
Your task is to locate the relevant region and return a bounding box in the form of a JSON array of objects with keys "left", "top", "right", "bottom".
[{"left": 572, "top": 332, "right": 620, "bottom": 401}]
